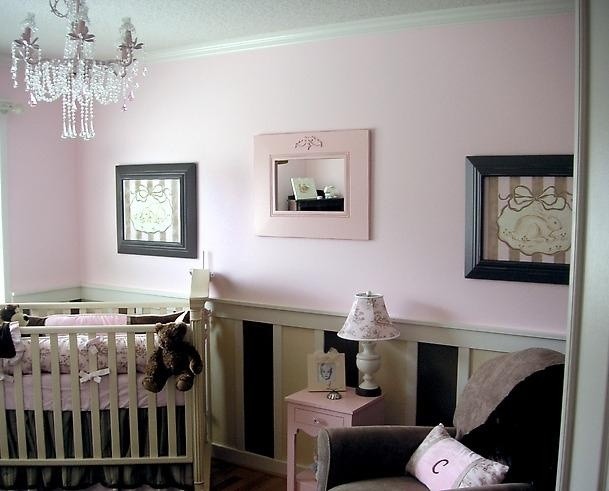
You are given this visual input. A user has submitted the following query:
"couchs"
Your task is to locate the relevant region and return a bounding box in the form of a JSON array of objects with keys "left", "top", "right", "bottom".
[{"left": 316, "top": 346, "right": 567, "bottom": 490}]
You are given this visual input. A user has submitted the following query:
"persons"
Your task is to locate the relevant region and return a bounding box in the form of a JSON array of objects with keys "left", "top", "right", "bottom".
[{"left": 320, "top": 364, "right": 332, "bottom": 380}]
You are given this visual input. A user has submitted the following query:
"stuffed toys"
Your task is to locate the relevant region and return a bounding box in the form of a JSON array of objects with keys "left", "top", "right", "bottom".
[{"left": 141, "top": 323, "right": 202, "bottom": 393}]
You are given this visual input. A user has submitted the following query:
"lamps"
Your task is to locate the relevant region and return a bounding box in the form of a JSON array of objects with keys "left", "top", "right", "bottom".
[
  {"left": 335, "top": 292, "right": 400, "bottom": 397},
  {"left": 9, "top": 0, "right": 147, "bottom": 144}
]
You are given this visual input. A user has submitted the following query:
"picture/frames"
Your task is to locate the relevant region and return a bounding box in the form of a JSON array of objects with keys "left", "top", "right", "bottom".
[
  {"left": 464, "top": 154, "right": 573, "bottom": 286},
  {"left": 307, "top": 350, "right": 346, "bottom": 392},
  {"left": 115, "top": 161, "right": 198, "bottom": 261}
]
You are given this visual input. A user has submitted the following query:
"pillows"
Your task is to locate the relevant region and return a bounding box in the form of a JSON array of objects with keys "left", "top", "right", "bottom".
[{"left": 403, "top": 424, "right": 508, "bottom": 490}]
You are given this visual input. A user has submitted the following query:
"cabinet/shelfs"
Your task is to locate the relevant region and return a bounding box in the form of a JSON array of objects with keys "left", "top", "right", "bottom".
[{"left": 283, "top": 385, "right": 384, "bottom": 490}]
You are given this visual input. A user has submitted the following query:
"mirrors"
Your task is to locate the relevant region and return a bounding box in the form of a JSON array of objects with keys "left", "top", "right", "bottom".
[{"left": 253, "top": 129, "right": 372, "bottom": 239}]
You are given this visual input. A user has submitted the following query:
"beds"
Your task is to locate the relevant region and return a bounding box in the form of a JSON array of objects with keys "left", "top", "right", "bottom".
[{"left": 1, "top": 267, "right": 213, "bottom": 489}]
